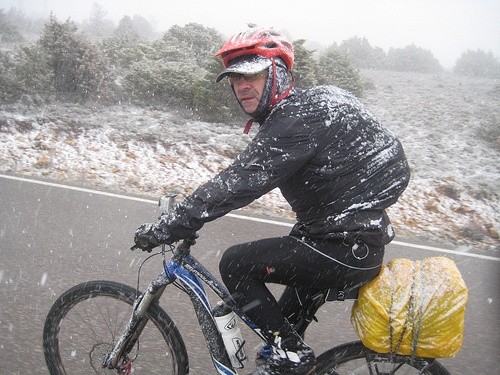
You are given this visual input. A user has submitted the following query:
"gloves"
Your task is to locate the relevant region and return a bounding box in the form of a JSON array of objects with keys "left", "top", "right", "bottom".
[{"left": 133, "top": 222, "right": 163, "bottom": 252}]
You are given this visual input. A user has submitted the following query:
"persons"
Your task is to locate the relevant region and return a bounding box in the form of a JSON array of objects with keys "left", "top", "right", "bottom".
[{"left": 133, "top": 29, "right": 410, "bottom": 375}]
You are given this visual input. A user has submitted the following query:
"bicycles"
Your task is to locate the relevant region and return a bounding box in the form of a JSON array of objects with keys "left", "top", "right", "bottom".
[{"left": 42, "top": 195, "right": 451, "bottom": 375}]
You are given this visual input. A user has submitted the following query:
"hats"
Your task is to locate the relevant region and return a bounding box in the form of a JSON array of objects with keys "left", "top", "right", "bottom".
[{"left": 216, "top": 53, "right": 288, "bottom": 83}]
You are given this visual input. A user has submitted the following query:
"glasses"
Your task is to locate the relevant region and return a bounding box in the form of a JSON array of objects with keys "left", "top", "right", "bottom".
[{"left": 225, "top": 69, "right": 268, "bottom": 82}]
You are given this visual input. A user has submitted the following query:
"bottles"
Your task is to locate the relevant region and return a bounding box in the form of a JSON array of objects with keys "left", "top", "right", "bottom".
[{"left": 211, "top": 301, "right": 247, "bottom": 368}]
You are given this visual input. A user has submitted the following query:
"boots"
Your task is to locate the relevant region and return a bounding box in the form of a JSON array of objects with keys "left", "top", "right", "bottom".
[{"left": 249, "top": 318, "right": 316, "bottom": 375}]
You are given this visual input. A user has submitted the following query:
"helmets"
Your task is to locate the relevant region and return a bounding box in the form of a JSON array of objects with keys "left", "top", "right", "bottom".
[{"left": 214, "top": 26, "right": 294, "bottom": 71}]
